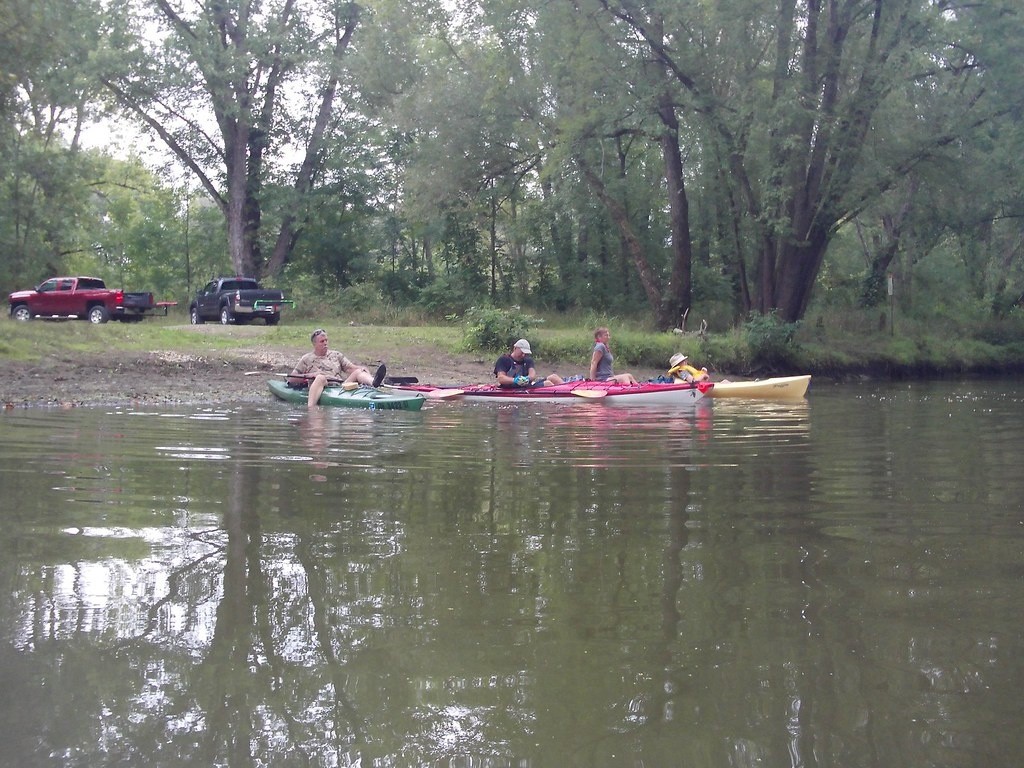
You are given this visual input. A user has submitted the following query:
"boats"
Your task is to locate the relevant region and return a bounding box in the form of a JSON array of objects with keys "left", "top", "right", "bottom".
[
  {"left": 267, "top": 380, "right": 426, "bottom": 411},
  {"left": 705, "top": 375, "right": 813, "bottom": 401},
  {"left": 384, "top": 382, "right": 714, "bottom": 408}
]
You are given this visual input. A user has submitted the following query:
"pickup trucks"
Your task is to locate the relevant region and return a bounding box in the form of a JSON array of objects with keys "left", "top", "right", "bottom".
[
  {"left": 8, "top": 277, "right": 153, "bottom": 324},
  {"left": 190, "top": 277, "right": 283, "bottom": 325}
]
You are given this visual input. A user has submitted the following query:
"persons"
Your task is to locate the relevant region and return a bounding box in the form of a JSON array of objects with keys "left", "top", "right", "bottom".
[
  {"left": 493, "top": 339, "right": 565, "bottom": 388},
  {"left": 669, "top": 353, "right": 708, "bottom": 383},
  {"left": 288, "top": 329, "right": 386, "bottom": 408},
  {"left": 590, "top": 328, "right": 638, "bottom": 386}
]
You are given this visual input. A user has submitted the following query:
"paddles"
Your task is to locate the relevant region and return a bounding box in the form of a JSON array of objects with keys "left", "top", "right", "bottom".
[
  {"left": 244, "top": 371, "right": 418, "bottom": 383},
  {"left": 428, "top": 388, "right": 608, "bottom": 399}
]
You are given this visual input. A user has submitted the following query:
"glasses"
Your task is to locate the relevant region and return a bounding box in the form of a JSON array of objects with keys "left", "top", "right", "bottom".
[{"left": 312, "top": 330, "right": 325, "bottom": 339}]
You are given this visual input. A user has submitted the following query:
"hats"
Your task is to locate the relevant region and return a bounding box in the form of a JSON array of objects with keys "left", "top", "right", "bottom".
[
  {"left": 669, "top": 353, "right": 688, "bottom": 367},
  {"left": 513, "top": 339, "right": 532, "bottom": 354}
]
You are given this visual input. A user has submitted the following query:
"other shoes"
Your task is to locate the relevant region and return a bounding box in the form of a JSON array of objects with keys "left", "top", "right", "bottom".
[{"left": 372, "top": 364, "right": 386, "bottom": 388}]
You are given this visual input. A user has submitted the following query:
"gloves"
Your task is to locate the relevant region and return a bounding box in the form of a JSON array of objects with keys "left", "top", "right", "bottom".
[{"left": 513, "top": 374, "right": 530, "bottom": 387}]
